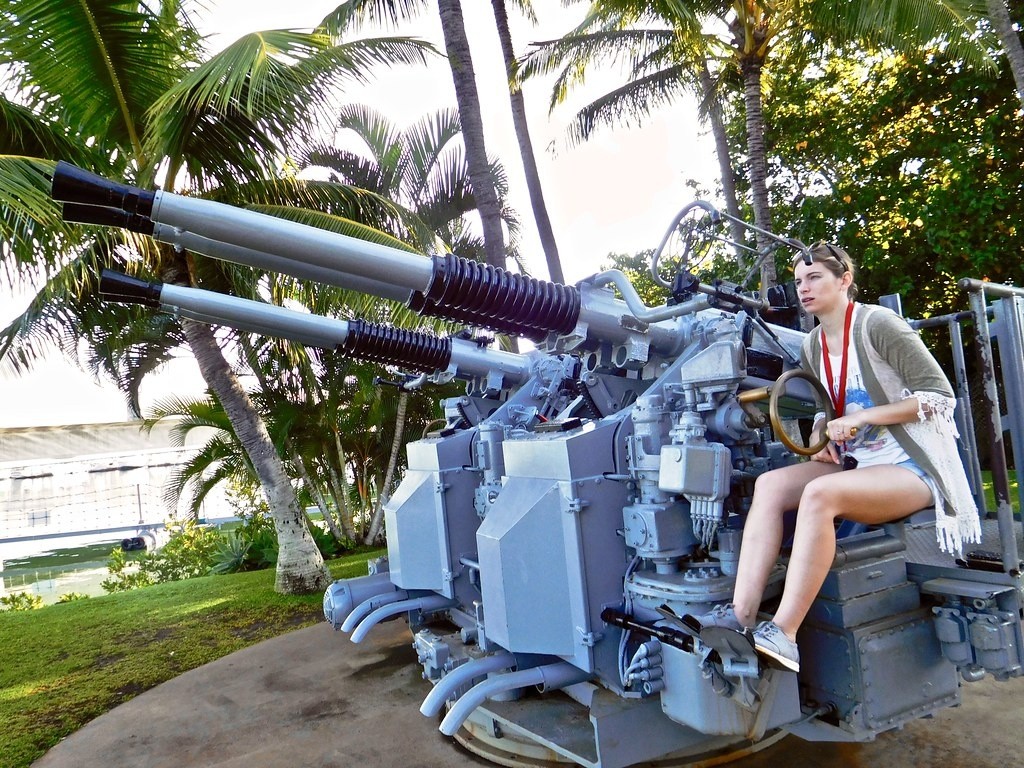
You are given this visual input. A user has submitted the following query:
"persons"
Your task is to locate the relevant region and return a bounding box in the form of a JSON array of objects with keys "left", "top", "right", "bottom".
[{"left": 678, "top": 239, "right": 982, "bottom": 672}]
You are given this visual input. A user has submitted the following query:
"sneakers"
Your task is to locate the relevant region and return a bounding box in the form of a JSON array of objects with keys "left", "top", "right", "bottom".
[
  {"left": 744, "top": 620, "right": 800, "bottom": 673},
  {"left": 684, "top": 603, "right": 756, "bottom": 634}
]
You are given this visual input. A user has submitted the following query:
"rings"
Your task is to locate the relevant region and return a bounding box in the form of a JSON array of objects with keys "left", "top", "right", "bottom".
[{"left": 837, "top": 430, "right": 844, "bottom": 434}]
